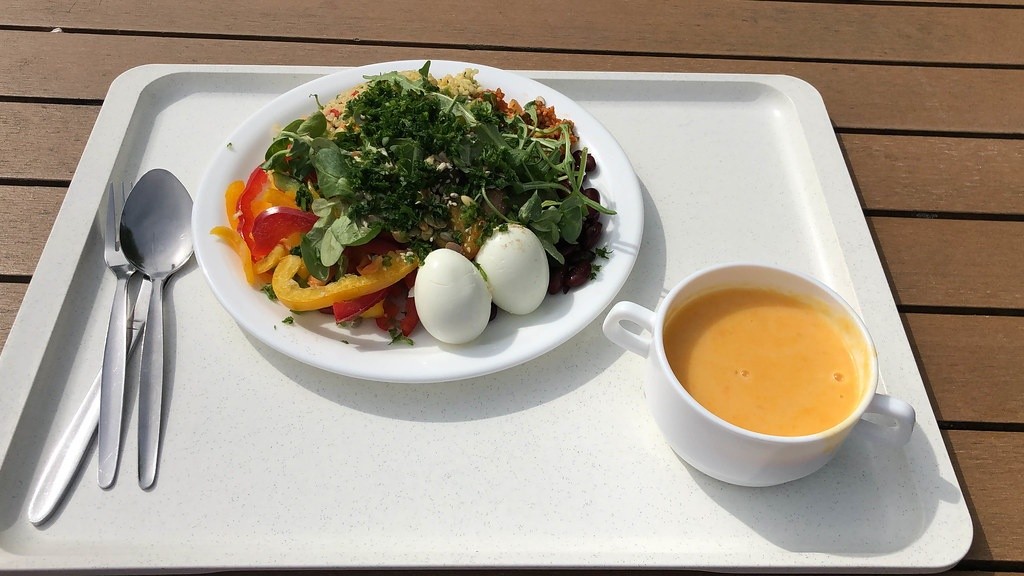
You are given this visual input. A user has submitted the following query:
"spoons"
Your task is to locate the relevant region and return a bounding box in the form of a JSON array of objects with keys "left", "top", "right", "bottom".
[{"left": 118, "top": 169, "right": 193, "bottom": 491}]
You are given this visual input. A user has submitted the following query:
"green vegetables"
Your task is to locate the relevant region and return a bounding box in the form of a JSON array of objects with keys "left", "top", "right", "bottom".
[{"left": 258, "top": 59, "right": 618, "bottom": 345}]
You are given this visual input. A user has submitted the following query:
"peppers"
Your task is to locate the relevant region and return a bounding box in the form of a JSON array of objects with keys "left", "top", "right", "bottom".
[{"left": 209, "top": 164, "right": 422, "bottom": 337}]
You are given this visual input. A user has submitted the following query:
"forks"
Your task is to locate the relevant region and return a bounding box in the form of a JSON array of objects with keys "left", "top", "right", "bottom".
[{"left": 97, "top": 182, "right": 138, "bottom": 489}]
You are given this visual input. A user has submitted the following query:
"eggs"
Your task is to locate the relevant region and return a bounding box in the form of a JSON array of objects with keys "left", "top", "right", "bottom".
[
  {"left": 414, "top": 248, "right": 492, "bottom": 344},
  {"left": 473, "top": 221, "right": 549, "bottom": 315}
]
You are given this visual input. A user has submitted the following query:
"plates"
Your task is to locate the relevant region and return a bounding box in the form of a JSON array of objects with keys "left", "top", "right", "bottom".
[{"left": 190, "top": 59, "right": 645, "bottom": 384}]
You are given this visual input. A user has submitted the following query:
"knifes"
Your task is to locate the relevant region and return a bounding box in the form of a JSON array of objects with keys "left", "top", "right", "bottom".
[{"left": 28, "top": 274, "right": 173, "bottom": 527}]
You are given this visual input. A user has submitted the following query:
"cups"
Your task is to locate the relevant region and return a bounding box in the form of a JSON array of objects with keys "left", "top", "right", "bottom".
[{"left": 602, "top": 259, "right": 915, "bottom": 489}]
